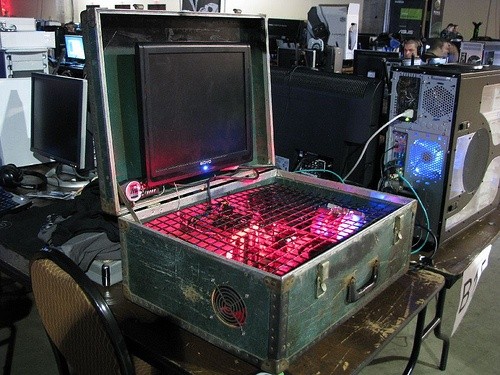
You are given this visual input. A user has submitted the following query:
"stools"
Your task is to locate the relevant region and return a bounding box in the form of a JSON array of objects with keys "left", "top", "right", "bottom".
[{"left": 0, "top": 281, "right": 33, "bottom": 375}]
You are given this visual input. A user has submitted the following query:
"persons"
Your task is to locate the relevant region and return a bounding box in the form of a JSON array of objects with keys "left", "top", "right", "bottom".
[
  {"left": 404, "top": 40, "right": 423, "bottom": 59},
  {"left": 421, "top": 38, "right": 450, "bottom": 58}
]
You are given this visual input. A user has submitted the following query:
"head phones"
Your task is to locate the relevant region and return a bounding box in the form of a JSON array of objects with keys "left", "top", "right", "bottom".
[
  {"left": 0, "top": 163, "right": 48, "bottom": 191},
  {"left": 401, "top": 37, "right": 424, "bottom": 56}
]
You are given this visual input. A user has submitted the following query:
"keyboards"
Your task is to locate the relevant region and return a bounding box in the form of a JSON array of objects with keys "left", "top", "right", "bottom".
[{"left": 1, "top": 192, "right": 32, "bottom": 216}]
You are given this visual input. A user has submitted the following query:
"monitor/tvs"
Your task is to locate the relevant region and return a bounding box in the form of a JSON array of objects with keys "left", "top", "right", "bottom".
[
  {"left": 64, "top": 34, "right": 86, "bottom": 60},
  {"left": 134, "top": 42, "right": 252, "bottom": 180},
  {"left": 353, "top": 49, "right": 401, "bottom": 80},
  {"left": 270, "top": 64, "right": 386, "bottom": 188},
  {"left": 30, "top": 73, "right": 99, "bottom": 189}
]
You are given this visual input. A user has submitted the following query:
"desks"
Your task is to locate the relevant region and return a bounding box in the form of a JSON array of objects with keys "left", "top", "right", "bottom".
[
  {"left": 0, "top": 161, "right": 446, "bottom": 375},
  {"left": 409, "top": 210, "right": 491, "bottom": 375}
]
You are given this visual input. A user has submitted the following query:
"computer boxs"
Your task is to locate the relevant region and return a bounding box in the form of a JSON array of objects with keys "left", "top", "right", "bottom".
[
  {"left": 384, "top": 62, "right": 500, "bottom": 249},
  {"left": 458, "top": 41, "right": 500, "bottom": 66}
]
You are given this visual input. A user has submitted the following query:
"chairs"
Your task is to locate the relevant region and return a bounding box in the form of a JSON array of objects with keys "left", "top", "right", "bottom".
[{"left": 28, "top": 248, "right": 153, "bottom": 375}]
